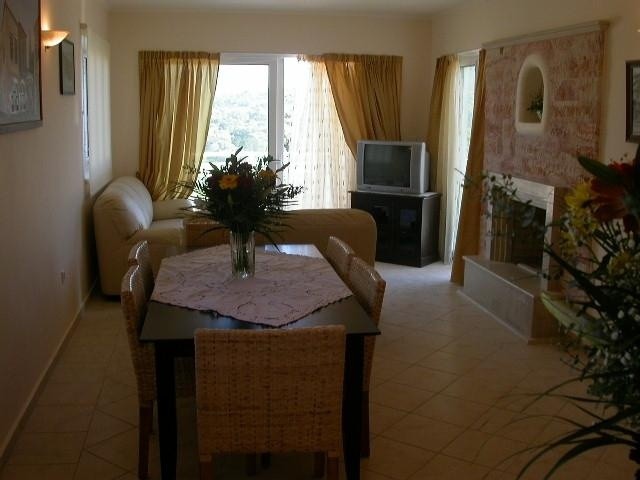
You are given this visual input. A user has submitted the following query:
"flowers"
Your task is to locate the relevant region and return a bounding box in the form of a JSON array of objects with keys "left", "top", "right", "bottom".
[
  {"left": 152, "top": 146, "right": 303, "bottom": 255},
  {"left": 471, "top": 148, "right": 640, "bottom": 480}
]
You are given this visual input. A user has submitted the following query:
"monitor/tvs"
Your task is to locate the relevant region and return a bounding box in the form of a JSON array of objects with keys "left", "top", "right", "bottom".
[{"left": 355, "top": 140, "right": 431, "bottom": 194}]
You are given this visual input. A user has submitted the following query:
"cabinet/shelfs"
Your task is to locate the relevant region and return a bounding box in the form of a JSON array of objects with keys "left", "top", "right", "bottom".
[{"left": 347, "top": 190, "right": 443, "bottom": 268}]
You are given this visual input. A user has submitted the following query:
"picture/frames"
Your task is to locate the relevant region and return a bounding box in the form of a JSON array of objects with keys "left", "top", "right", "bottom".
[
  {"left": 0, "top": 0, "right": 43, "bottom": 134},
  {"left": 59, "top": 38, "right": 75, "bottom": 96},
  {"left": 625, "top": 59, "right": 640, "bottom": 144}
]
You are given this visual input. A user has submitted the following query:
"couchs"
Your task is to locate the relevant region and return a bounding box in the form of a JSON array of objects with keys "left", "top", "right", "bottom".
[{"left": 91, "top": 176, "right": 378, "bottom": 303}]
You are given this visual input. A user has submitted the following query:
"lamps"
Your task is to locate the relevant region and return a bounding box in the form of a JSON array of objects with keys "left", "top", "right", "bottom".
[{"left": 41, "top": 29, "right": 70, "bottom": 47}]
